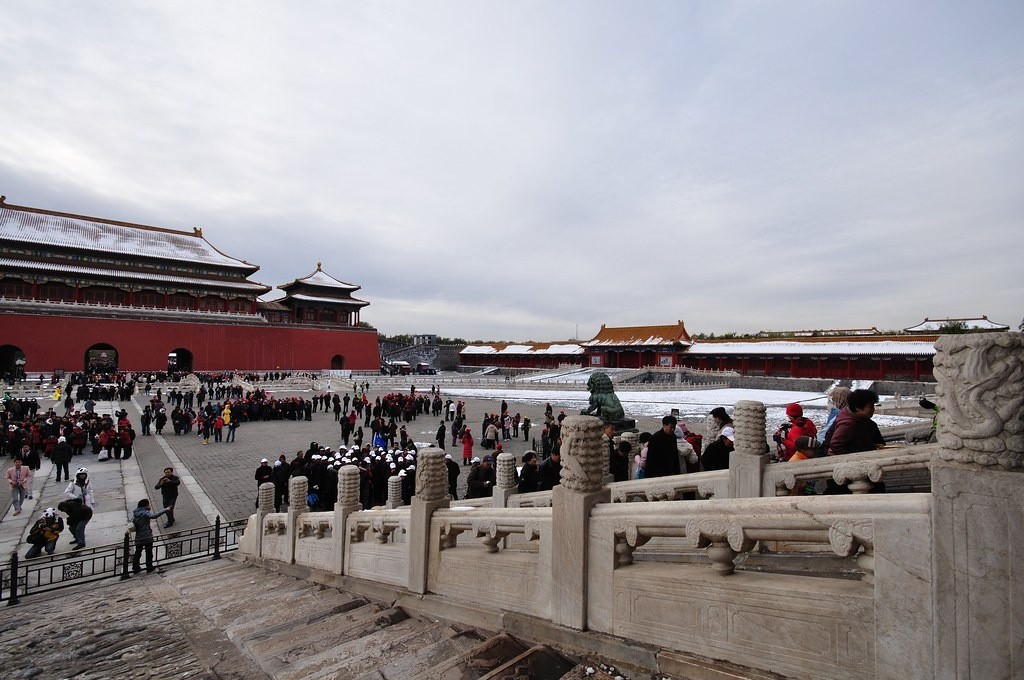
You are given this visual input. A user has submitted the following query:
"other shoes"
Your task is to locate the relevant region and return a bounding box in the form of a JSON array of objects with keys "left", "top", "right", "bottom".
[
  {"left": 504, "top": 438, "right": 510, "bottom": 441},
  {"left": 69, "top": 540, "right": 77, "bottom": 544},
  {"left": 72, "top": 545, "right": 85, "bottom": 550},
  {"left": 25, "top": 495, "right": 32, "bottom": 499},
  {"left": 13, "top": 511, "right": 21, "bottom": 515},
  {"left": 48, "top": 551, "right": 54, "bottom": 554},
  {"left": 207, "top": 438, "right": 210, "bottom": 443},
  {"left": 163, "top": 519, "right": 176, "bottom": 529},
  {"left": 202, "top": 439, "right": 208, "bottom": 445}
]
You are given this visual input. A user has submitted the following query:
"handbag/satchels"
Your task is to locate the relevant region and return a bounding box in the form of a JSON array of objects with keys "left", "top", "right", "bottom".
[
  {"left": 127, "top": 522, "right": 136, "bottom": 532},
  {"left": 232, "top": 421, "right": 240, "bottom": 428},
  {"left": 634, "top": 461, "right": 643, "bottom": 479},
  {"left": 97, "top": 447, "right": 108, "bottom": 460},
  {"left": 481, "top": 437, "right": 488, "bottom": 447}
]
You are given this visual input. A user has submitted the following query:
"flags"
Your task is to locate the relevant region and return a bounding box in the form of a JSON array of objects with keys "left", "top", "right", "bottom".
[{"left": 373, "top": 434, "right": 386, "bottom": 448}]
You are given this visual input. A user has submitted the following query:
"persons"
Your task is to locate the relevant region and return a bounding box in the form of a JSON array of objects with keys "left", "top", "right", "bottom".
[
  {"left": 25, "top": 507, "right": 64, "bottom": 559},
  {"left": 132, "top": 499, "right": 173, "bottom": 573},
  {"left": 602, "top": 406, "right": 734, "bottom": 499},
  {"left": 155, "top": 468, "right": 180, "bottom": 528},
  {"left": 919, "top": 396, "right": 939, "bottom": 444},
  {"left": 772, "top": 386, "right": 887, "bottom": 494},
  {"left": 64, "top": 467, "right": 95, "bottom": 508},
  {"left": 254, "top": 442, "right": 461, "bottom": 513},
  {"left": 18, "top": 443, "right": 41, "bottom": 500},
  {"left": 51, "top": 436, "right": 73, "bottom": 483},
  {"left": 57, "top": 498, "right": 93, "bottom": 551},
  {"left": 6, "top": 458, "right": 31, "bottom": 515},
  {"left": 462, "top": 400, "right": 568, "bottom": 499},
  {"left": 0, "top": 361, "right": 466, "bottom": 465}
]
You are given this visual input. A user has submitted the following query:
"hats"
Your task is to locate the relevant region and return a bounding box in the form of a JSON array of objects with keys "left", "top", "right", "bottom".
[
  {"left": 720, "top": 427, "right": 735, "bottom": 441},
  {"left": 73, "top": 467, "right": 89, "bottom": 484},
  {"left": 445, "top": 453, "right": 453, "bottom": 459},
  {"left": 490, "top": 411, "right": 528, "bottom": 418},
  {"left": 311, "top": 443, "right": 416, "bottom": 477},
  {"left": 261, "top": 458, "right": 269, "bottom": 463},
  {"left": 458, "top": 400, "right": 461, "bottom": 403},
  {"left": 58, "top": 436, "right": 66, "bottom": 444},
  {"left": 543, "top": 429, "right": 548, "bottom": 433},
  {"left": 786, "top": 403, "right": 803, "bottom": 416},
  {"left": 275, "top": 460, "right": 282, "bottom": 467},
  {"left": 465, "top": 429, "right": 471, "bottom": 432},
  {"left": 794, "top": 436, "right": 821, "bottom": 451},
  {"left": 673, "top": 423, "right": 688, "bottom": 438},
  {"left": 470, "top": 444, "right": 503, "bottom": 465}
]
